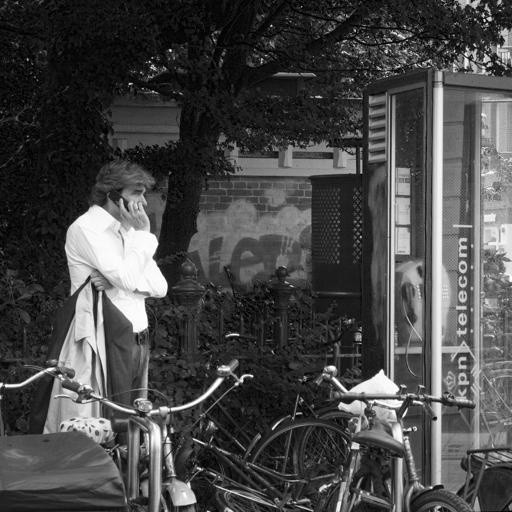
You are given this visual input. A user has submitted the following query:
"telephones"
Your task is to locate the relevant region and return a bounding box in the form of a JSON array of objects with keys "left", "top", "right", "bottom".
[{"left": 394, "top": 260, "right": 451, "bottom": 344}]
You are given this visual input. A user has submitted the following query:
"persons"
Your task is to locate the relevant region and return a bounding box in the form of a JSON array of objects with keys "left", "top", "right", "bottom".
[{"left": 42, "top": 160, "right": 169, "bottom": 496}]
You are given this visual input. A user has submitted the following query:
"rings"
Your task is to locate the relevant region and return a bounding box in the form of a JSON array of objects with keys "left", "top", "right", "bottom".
[
  {"left": 134, "top": 207, "right": 138, "bottom": 210},
  {"left": 119, "top": 214, "right": 122, "bottom": 217}
]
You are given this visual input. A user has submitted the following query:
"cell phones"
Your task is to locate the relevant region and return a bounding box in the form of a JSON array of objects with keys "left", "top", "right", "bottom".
[{"left": 108, "top": 187, "right": 129, "bottom": 213}]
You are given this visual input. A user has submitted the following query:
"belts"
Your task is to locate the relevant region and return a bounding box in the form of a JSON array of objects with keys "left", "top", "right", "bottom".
[{"left": 134, "top": 328, "right": 149, "bottom": 345}]
